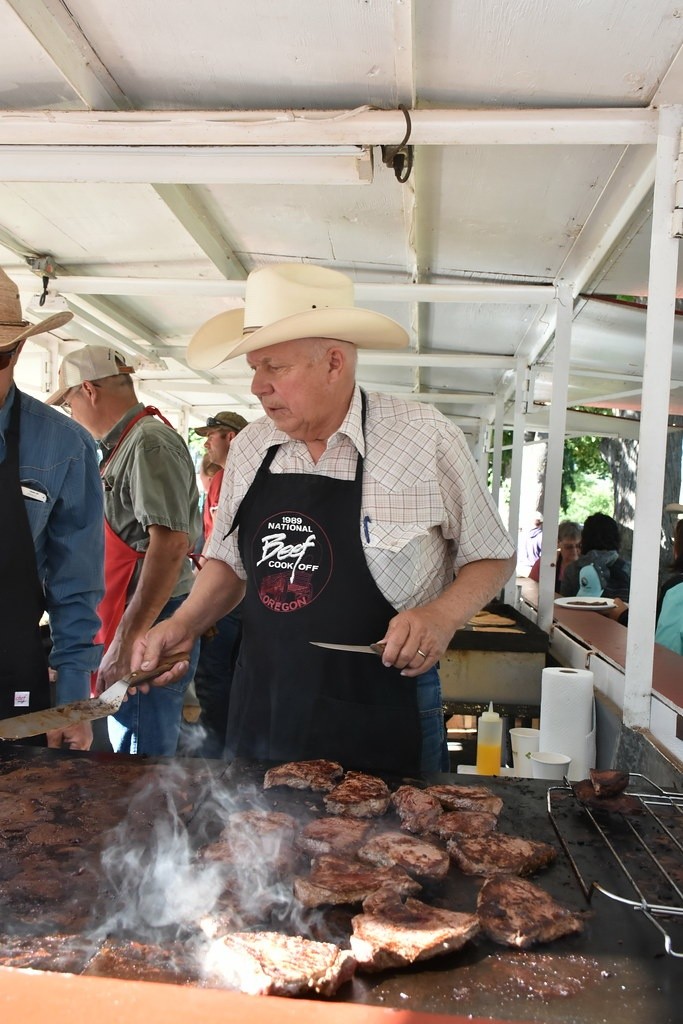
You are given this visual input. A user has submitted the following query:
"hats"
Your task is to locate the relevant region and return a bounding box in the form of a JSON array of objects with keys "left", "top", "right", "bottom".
[
  {"left": 582, "top": 512, "right": 618, "bottom": 545},
  {"left": 185, "top": 262, "right": 409, "bottom": 370},
  {"left": 44, "top": 344, "right": 135, "bottom": 406},
  {"left": 0, "top": 267, "right": 74, "bottom": 348},
  {"left": 194, "top": 412, "right": 248, "bottom": 437}
]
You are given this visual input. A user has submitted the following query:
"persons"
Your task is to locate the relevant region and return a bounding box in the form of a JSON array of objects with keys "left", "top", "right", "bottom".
[
  {"left": 529, "top": 521, "right": 584, "bottom": 595},
  {"left": 561, "top": 513, "right": 631, "bottom": 597},
  {"left": 525, "top": 519, "right": 542, "bottom": 568},
  {"left": 42, "top": 343, "right": 201, "bottom": 758},
  {"left": 595, "top": 520, "right": 683, "bottom": 658},
  {"left": 124, "top": 259, "right": 518, "bottom": 779},
  {"left": 191, "top": 410, "right": 249, "bottom": 576},
  {"left": 0, "top": 266, "right": 106, "bottom": 751}
]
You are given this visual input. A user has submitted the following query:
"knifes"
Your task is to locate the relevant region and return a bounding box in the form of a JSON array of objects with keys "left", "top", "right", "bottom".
[{"left": 307, "top": 641, "right": 386, "bottom": 656}]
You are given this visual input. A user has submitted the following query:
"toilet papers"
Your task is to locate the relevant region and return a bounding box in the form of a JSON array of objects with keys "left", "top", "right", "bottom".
[{"left": 538, "top": 666, "right": 598, "bottom": 781}]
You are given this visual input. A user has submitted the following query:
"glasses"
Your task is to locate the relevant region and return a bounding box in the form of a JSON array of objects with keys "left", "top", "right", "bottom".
[
  {"left": 60, "top": 384, "right": 101, "bottom": 415},
  {"left": 561, "top": 543, "right": 581, "bottom": 551},
  {"left": 0, "top": 341, "right": 21, "bottom": 371},
  {"left": 206, "top": 417, "right": 240, "bottom": 432}
]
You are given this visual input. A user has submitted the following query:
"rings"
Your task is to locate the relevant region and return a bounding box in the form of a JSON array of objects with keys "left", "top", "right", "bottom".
[{"left": 417, "top": 649, "right": 426, "bottom": 658}]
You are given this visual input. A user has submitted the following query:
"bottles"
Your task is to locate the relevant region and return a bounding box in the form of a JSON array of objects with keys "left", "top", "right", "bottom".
[{"left": 476, "top": 700, "right": 502, "bottom": 776}]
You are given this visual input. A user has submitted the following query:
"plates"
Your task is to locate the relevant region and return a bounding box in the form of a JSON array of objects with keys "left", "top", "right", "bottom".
[{"left": 554, "top": 596, "right": 616, "bottom": 609}]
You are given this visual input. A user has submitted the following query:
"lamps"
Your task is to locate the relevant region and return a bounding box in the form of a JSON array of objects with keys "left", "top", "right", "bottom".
[
  {"left": 0, "top": 144, "right": 378, "bottom": 186},
  {"left": 25, "top": 287, "right": 169, "bottom": 373}
]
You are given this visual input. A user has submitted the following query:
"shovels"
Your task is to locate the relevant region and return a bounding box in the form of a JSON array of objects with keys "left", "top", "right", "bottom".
[{"left": 0, "top": 651, "right": 191, "bottom": 742}]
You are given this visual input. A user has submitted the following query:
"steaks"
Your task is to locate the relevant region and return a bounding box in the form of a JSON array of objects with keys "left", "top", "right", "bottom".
[
  {"left": 566, "top": 601, "right": 607, "bottom": 606},
  {"left": 572, "top": 767, "right": 645, "bottom": 814},
  {"left": 1, "top": 759, "right": 578, "bottom": 999}
]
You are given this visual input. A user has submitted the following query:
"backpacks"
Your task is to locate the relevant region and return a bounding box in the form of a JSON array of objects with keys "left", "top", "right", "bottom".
[{"left": 577, "top": 557, "right": 630, "bottom": 626}]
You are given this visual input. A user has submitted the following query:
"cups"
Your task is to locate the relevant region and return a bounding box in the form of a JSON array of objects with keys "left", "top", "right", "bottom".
[
  {"left": 509, "top": 727, "right": 540, "bottom": 778},
  {"left": 530, "top": 752, "right": 573, "bottom": 780}
]
